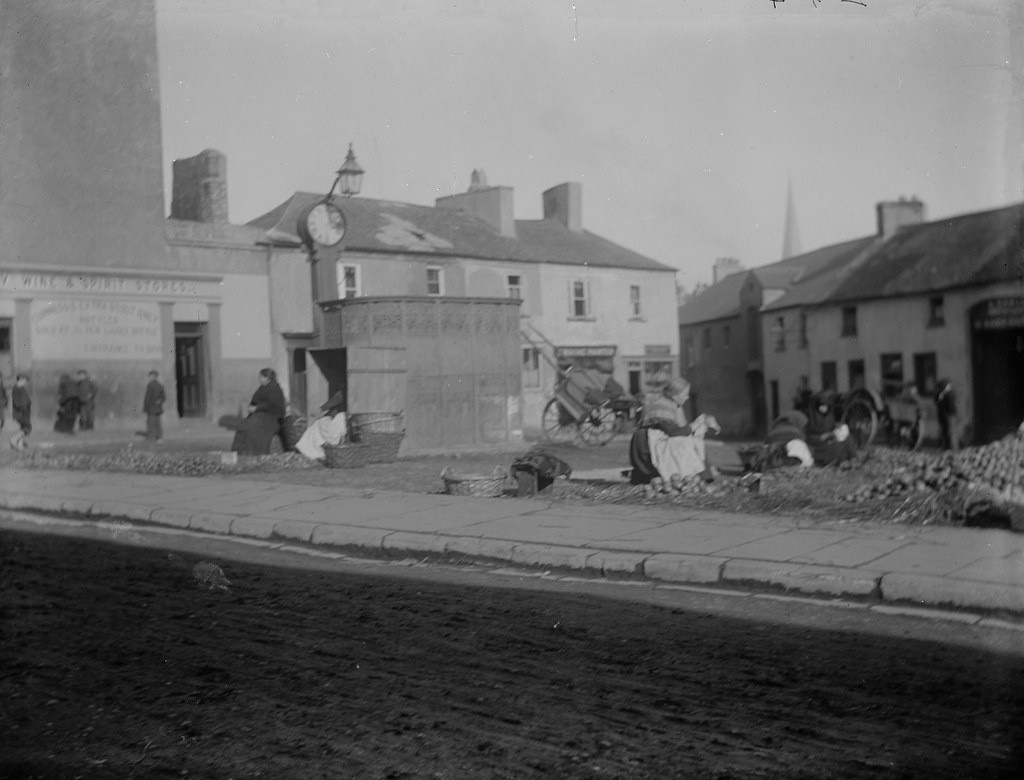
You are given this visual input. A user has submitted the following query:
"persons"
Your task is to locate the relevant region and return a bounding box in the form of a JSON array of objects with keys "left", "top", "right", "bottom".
[
  {"left": 215, "top": 400, "right": 282, "bottom": 455},
  {"left": 933, "top": 379, "right": 961, "bottom": 453},
  {"left": 641, "top": 370, "right": 670, "bottom": 425},
  {"left": 75, "top": 369, "right": 99, "bottom": 432},
  {"left": 630, "top": 377, "right": 723, "bottom": 486},
  {"left": 58, "top": 372, "right": 86, "bottom": 433},
  {"left": 142, "top": 370, "right": 165, "bottom": 444},
  {"left": 0, "top": 371, "right": 8, "bottom": 432},
  {"left": 804, "top": 388, "right": 858, "bottom": 466},
  {"left": 247, "top": 368, "right": 286, "bottom": 436},
  {"left": 10, "top": 373, "right": 33, "bottom": 453}
]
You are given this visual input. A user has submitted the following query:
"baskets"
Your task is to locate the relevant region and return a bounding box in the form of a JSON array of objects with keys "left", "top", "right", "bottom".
[
  {"left": 440, "top": 465, "right": 509, "bottom": 498},
  {"left": 282, "top": 406, "right": 405, "bottom": 469}
]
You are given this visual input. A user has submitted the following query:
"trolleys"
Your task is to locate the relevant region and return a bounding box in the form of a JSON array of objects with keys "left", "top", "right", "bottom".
[{"left": 519, "top": 315, "right": 646, "bottom": 448}]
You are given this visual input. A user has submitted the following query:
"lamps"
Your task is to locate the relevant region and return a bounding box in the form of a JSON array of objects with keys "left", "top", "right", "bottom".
[{"left": 328, "top": 141, "right": 365, "bottom": 201}]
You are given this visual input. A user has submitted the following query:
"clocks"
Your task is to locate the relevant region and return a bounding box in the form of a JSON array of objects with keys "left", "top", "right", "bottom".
[{"left": 307, "top": 205, "right": 346, "bottom": 245}]
